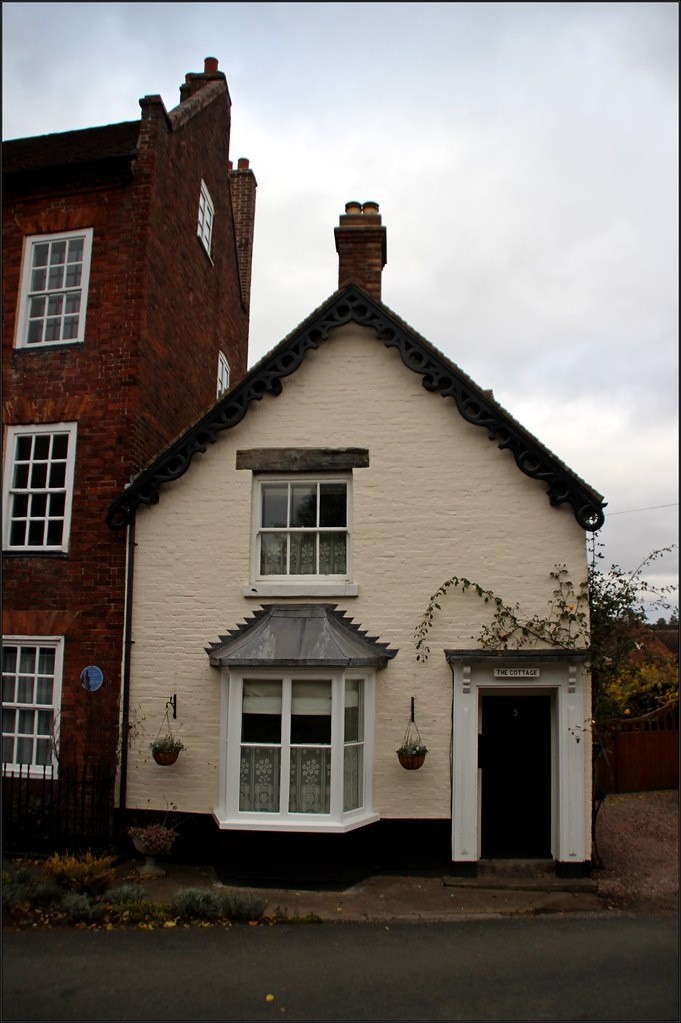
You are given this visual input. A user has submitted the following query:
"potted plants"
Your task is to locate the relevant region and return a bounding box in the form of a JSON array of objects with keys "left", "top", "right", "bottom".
[
  {"left": 396, "top": 718, "right": 428, "bottom": 771},
  {"left": 150, "top": 708, "right": 184, "bottom": 765}
]
